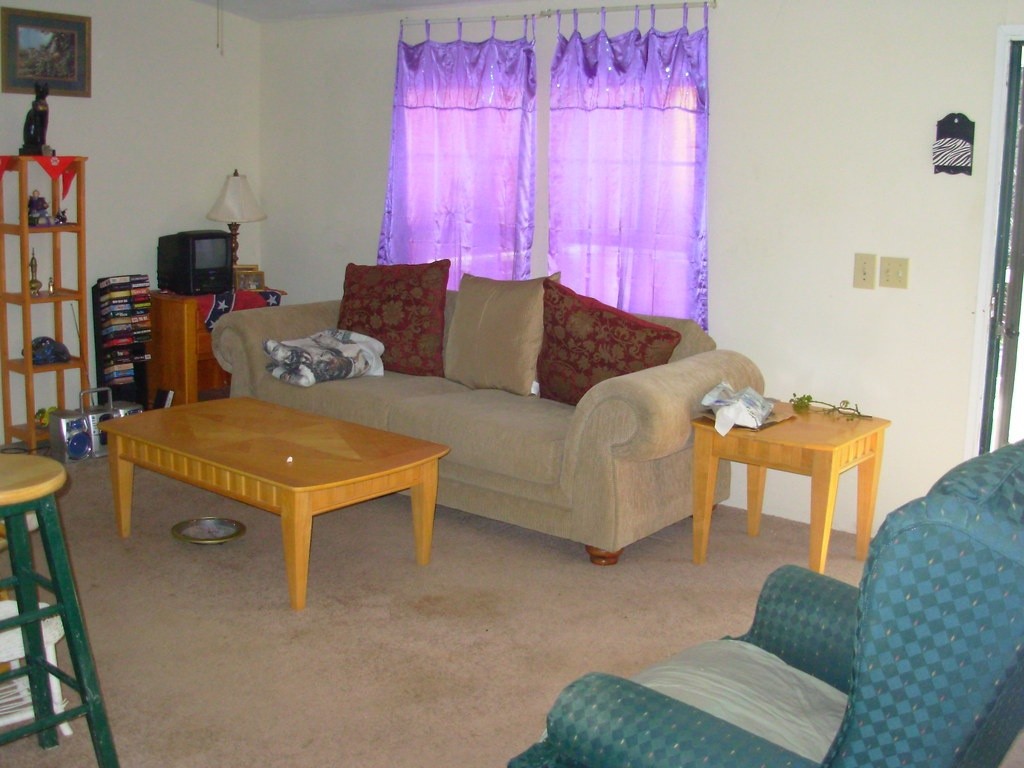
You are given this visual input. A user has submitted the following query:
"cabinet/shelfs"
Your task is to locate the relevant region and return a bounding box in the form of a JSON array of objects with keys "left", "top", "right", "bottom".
[
  {"left": 92, "top": 275, "right": 145, "bottom": 443},
  {"left": 146, "top": 289, "right": 280, "bottom": 411},
  {"left": 0, "top": 155, "right": 90, "bottom": 455}
]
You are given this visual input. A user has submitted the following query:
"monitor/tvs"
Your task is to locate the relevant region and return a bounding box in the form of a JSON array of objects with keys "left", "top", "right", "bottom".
[{"left": 157, "top": 230, "right": 233, "bottom": 295}]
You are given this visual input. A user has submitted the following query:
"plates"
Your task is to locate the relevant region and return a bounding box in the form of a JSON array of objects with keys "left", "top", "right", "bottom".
[{"left": 171, "top": 516, "right": 246, "bottom": 544}]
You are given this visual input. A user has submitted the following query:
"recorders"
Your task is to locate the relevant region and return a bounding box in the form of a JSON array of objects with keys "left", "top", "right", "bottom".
[{"left": 49, "top": 400, "right": 143, "bottom": 464}]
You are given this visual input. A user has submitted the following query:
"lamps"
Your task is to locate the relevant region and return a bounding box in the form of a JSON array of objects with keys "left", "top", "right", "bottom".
[{"left": 207, "top": 169, "right": 267, "bottom": 264}]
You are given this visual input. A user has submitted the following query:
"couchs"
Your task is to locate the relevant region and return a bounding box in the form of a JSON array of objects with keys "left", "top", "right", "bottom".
[
  {"left": 508, "top": 441, "right": 1024, "bottom": 768},
  {"left": 211, "top": 301, "right": 763, "bottom": 563}
]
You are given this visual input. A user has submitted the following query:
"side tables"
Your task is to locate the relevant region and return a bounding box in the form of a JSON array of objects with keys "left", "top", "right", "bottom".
[{"left": 692, "top": 398, "right": 893, "bottom": 574}]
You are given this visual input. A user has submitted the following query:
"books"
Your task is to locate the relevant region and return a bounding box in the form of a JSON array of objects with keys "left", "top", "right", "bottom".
[
  {"left": 100, "top": 276, "right": 153, "bottom": 387},
  {"left": 150, "top": 387, "right": 173, "bottom": 410}
]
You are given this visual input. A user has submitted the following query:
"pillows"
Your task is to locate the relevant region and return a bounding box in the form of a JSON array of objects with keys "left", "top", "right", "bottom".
[
  {"left": 337, "top": 259, "right": 452, "bottom": 374},
  {"left": 444, "top": 269, "right": 685, "bottom": 407}
]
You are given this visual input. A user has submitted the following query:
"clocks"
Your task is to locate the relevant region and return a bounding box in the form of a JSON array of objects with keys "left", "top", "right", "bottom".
[{"left": 172, "top": 517, "right": 247, "bottom": 546}]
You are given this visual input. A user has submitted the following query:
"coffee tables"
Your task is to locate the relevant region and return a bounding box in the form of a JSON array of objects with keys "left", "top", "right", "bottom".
[{"left": 106, "top": 401, "right": 447, "bottom": 610}]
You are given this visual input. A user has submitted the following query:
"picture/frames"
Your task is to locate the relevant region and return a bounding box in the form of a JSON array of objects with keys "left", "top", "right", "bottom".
[
  {"left": 234, "top": 265, "right": 266, "bottom": 290},
  {"left": 0, "top": 7, "right": 92, "bottom": 98}
]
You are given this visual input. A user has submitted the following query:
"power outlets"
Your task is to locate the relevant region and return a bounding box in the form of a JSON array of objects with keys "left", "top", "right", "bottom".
[{"left": 852, "top": 254, "right": 877, "bottom": 289}]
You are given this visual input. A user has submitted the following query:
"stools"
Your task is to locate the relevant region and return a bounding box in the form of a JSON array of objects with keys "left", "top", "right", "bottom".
[{"left": 0, "top": 454, "right": 124, "bottom": 768}]
